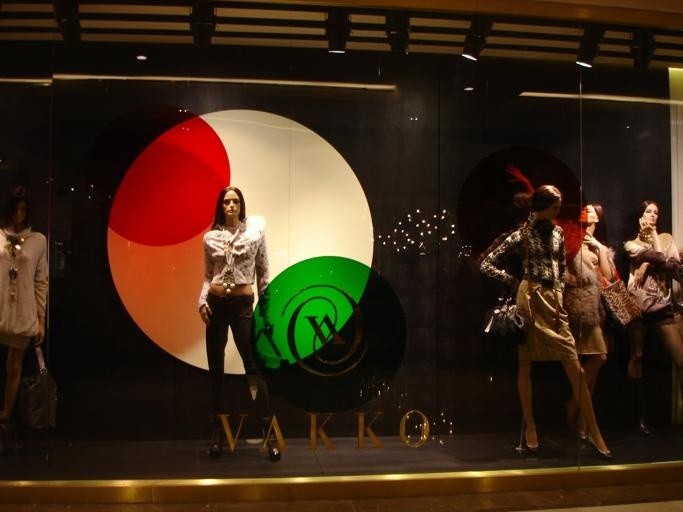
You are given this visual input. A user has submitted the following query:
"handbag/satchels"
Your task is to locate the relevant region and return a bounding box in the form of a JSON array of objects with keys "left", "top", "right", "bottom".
[
  {"left": 482, "top": 294, "right": 530, "bottom": 344},
  {"left": 601, "top": 278, "right": 644, "bottom": 327},
  {"left": 16, "top": 365, "right": 58, "bottom": 429}
]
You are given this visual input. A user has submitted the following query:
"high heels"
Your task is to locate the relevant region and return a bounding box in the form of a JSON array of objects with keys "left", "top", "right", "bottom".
[
  {"left": 525, "top": 427, "right": 539, "bottom": 453},
  {"left": 209, "top": 429, "right": 223, "bottom": 458},
  {"left": 588, "top": 432, "right": 613, "bottom": 460},
  {"left": 267, "top": 441, "right": 279, "bottom": 461},
  {"left": 576, "top": 415, "right": 587, "bottom": 441},
  {"left": 565, "top": 400, "right": 576, "bottom": 425}
]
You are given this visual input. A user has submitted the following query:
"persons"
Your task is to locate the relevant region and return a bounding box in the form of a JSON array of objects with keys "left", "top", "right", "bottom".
[
  {"left": 0, "top": 186, "right": 55, "bottom": 421},
  {"left": 198, "top": 187, "right": 280, "bottom": 461},
  {"left": 506, "top": 165, "right": 622, "bottom": 442},
  {"left": 623, "top": 200, "right": 683, "bottom": 437},
  {"left": 479, "top": 184, "right": 615, "bottom": 459}
]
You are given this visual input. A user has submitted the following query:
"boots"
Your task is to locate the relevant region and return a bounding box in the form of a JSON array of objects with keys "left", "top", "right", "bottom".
[{"left": 628, "top": 377, "right": 651, "bottom": 435}]
